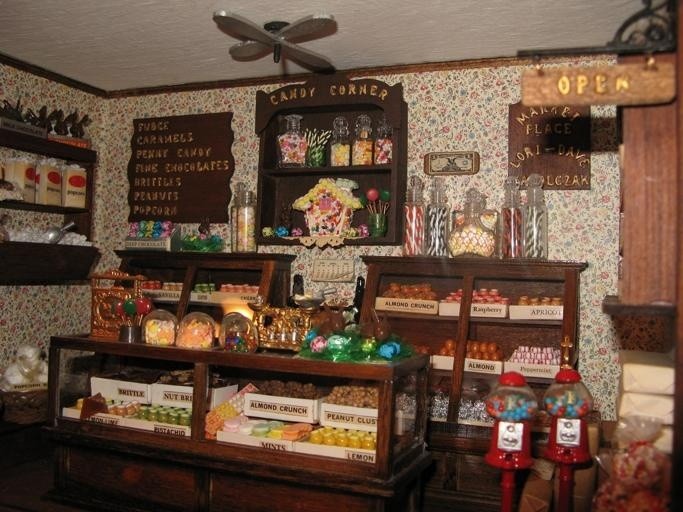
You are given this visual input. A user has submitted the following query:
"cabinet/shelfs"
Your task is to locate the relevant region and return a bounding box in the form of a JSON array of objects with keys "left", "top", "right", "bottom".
[
  {"left": 0, "top": 127, "right": 99, "bottom": 285},
  {"left": 254, "top": 79, "right": 408, "bottom": 246},
  {"left": 112, "top": 250, "right": 295, "bottom": 350},
  {"left": 42, "top": 333, "right": 438, "bottom": 512},
  {"left": 356, "top": 254, "right": 588, "bottom": 511}
]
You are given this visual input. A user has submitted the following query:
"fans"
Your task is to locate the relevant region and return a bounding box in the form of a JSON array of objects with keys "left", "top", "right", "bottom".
[{"left": 211, "top": 11, "right": 338, "bottom": 75}]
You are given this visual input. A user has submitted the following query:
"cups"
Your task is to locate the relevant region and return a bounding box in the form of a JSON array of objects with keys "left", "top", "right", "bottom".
[{"left": 367, "top": 213, "right": 389, "bottom": 239}]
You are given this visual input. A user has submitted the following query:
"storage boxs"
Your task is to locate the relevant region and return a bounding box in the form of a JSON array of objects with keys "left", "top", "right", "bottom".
[
  {"left": 319, "top": 400, "right": 417, "bottom": 436},
  {"left": 90, "top": 373, "right": 151, "bottom": 408},
  {"left": 148, "top": 380, "right": 239, "bottom": 413},
  {"left": 244, "top": 393, "right": 325, "bottom": 424}
]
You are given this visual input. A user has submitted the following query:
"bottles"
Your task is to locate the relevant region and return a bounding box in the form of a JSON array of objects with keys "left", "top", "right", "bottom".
[
  {"left": 402, "top": 173, "right": 548, "bottom": 260},
  {"left": 236, "top": 191, "right": 257, "bottom": 253},
  {"left": 276, "top": 114, "right": 392, "bottom": 168},
  {"left": 230, "top": 182, "right": 245, "bottom": 253},
  {"left": 141, "top": 281, "right": 258, "bottom": 294}
]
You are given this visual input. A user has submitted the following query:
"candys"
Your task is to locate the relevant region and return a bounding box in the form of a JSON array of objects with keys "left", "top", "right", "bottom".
[
  {"left": 485, "top": 392, "right": 537, "bottom": 420},
  {"left": 544, "top": 394, "right": 586, "bottom": 418}
]
